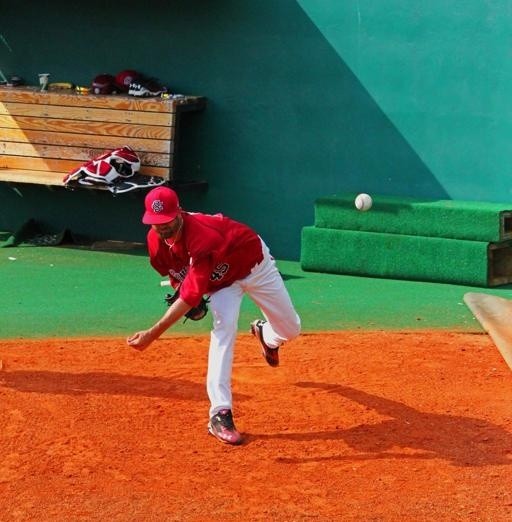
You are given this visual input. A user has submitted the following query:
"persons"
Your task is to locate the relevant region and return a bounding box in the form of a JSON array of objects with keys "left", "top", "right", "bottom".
[{"left": 126, "top": 186, "right": 301, "bottom": 445}]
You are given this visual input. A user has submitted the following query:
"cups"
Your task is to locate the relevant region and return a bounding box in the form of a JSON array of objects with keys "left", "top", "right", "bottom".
[{"left": 37, "top": 73, "right": 51, "bottom": 93}]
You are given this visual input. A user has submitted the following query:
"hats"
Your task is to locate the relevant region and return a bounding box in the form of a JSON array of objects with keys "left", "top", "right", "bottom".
[{"left": 141, "top": 185, "right": 181, "bottom": 226}]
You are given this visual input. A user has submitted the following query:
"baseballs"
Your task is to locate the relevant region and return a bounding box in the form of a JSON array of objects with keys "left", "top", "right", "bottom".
[{"left": 355, "top": 194, "right": 372, "bottom": 210}]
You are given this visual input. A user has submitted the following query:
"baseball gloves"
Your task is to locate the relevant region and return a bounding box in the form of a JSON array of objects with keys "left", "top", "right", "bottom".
[{"left": 165, "top": 284, "right": 209, "bottom": 321}]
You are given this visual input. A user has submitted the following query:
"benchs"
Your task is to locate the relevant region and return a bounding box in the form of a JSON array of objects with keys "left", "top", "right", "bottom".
[
  {"left": 300, "top": 193, "right": 512, "bottom": 287},
  {"left": 0, "top": 85, "right": 209, "bottom": 194}
]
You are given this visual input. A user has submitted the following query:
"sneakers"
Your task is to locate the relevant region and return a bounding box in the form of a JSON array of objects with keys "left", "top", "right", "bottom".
[
  {"left": 127, "top": 79, "right": 162, "bottom": 97},
  {"left": 250, "top": 318, "right": 280, "bottom": 368},
  {"left": 207, "top": 409, "right": 242, "bottom": 445}
]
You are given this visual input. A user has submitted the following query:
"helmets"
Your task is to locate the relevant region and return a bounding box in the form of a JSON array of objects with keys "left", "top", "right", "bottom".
[
  {"left": 91, "top": 73, "right": 115, "bottom": 95},
  {"left": 116, "top": 71, "right": 138, "bottom": 93}
]
